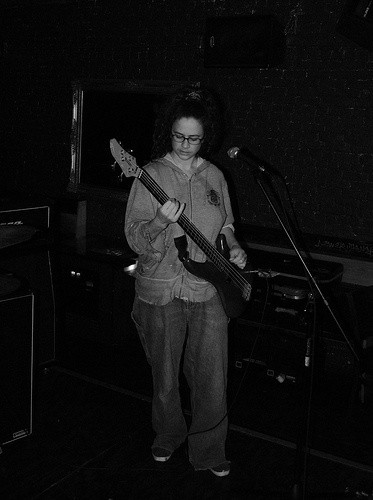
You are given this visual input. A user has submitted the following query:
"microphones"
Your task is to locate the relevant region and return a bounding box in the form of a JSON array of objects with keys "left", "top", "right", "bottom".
[{"left": 227, "top": 146, "right": 265, "bottom": 172}]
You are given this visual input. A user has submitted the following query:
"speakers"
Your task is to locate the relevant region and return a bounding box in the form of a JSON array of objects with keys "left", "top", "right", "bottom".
[{"left": 0, "top": 272, "right": 38, "bottom": 447}]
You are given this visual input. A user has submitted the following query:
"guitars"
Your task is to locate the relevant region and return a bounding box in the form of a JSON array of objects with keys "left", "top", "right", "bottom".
[{"left": 107, "top": 138, "right": 258, "bottom": 320}]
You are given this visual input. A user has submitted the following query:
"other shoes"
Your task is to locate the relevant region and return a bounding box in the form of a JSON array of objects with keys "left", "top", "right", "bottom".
[
  {"left": 151, "top": 447, "right": 172, "bottom": 462},
  {"left": 209, "top": 460, "right": 230, "bottom": 476}
]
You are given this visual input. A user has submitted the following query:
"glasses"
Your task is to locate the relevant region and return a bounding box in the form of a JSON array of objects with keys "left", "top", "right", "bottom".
[{"left": 170, "top": 132, "right": 203, "bottom": 145}]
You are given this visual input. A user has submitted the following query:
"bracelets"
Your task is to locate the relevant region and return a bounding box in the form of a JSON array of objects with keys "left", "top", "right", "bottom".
[{"left": 146, "top": 230, "right": 152, "bottom": 242}]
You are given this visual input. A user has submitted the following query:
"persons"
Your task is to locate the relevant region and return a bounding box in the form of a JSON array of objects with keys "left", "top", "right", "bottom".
[{"left": 124, "top": 99, "right": 247, "bottom": 476}]
